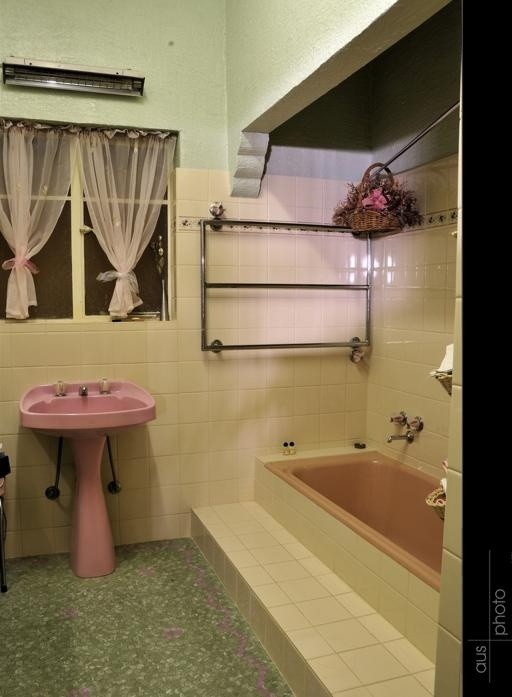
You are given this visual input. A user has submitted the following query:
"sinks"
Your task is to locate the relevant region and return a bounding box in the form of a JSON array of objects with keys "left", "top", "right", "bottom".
[{"left": 19, "top": 377, "right": 156, "bottom": 436}]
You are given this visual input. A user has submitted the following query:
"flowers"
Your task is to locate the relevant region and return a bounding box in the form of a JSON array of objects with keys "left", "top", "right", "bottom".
[{"left": 333, "top": 160, "right": 423, "bottom": 234}]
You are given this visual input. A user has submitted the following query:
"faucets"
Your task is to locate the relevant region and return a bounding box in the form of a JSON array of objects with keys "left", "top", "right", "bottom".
[
  {"left": 79, "top": 385, "right": 88, "bottom": 397},
  {"left": 387, "top": 430, "right": 415, "bottom": 444}
]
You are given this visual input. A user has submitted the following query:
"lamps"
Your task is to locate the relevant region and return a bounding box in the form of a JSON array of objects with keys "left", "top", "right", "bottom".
[{"left": 2, "top": 55, "right": 147, "bottom": 101}]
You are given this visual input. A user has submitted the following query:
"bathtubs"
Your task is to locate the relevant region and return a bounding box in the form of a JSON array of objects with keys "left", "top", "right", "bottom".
[{"left": 263, "top": 451, "right": 446, "bottom": 592}]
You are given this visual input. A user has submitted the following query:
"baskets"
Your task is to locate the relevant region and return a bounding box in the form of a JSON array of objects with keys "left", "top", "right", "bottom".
[{"left": 344, "top": 208, "right": 401, "bottom": 232}]
